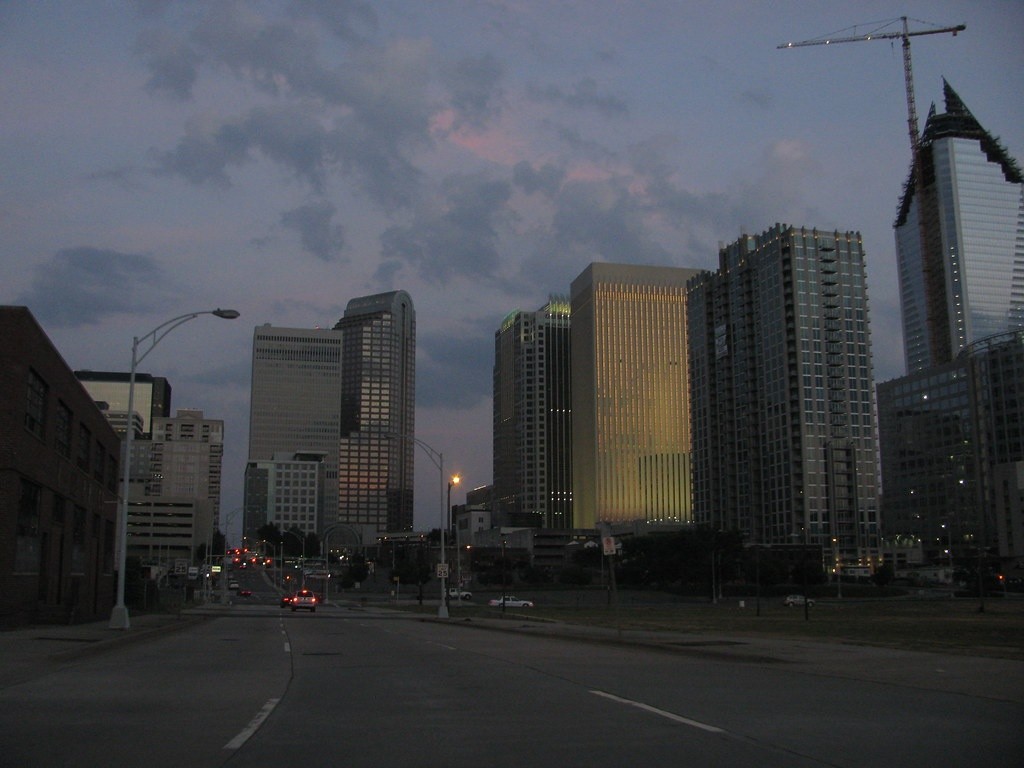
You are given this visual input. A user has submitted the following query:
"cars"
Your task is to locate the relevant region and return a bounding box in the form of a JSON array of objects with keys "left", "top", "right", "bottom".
[
  {"left": 225, "top": 548, "right": 272, "bottom": 598},
  {"left": 280, "top": 593, "right": 294, "bottom": 608}
]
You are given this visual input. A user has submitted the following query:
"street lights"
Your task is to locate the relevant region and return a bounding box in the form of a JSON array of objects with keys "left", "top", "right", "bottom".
[
  {"left": 107, "top": 307, "right": 241, "bottom": 630},
  {"left": 446, "top": 475, "right": 460, "bottom": 607},
  {"left": 384, "top": 431, "right": 449, "bottom": 619}
]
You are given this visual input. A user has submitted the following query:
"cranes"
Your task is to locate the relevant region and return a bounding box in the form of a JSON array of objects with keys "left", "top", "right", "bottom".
[{"left": 775, "top": 14, "right": 966, "bottom": 363}]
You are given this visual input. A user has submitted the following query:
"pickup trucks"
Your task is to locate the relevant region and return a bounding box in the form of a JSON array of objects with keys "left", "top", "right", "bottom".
[
  {"left": 292, "top": 590, "right": 315, "bottom": 612},
  {"left": 489, "top": 596, "right": 533, "bottom": 607}
]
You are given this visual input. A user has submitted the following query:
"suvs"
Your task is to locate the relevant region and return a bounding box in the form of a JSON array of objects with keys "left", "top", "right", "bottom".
[{"left": 444, "top": 588, "right": 472, "bottom": 600}]
